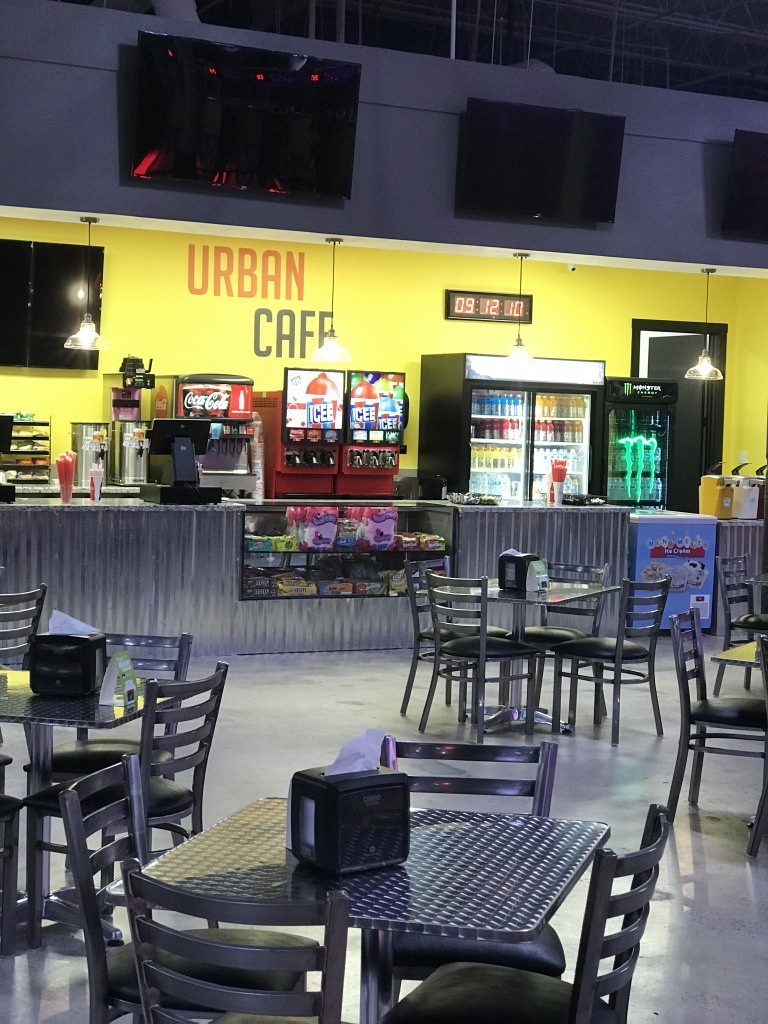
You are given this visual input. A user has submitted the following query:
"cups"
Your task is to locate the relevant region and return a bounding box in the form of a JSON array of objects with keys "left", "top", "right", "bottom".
[
  {"left": 304, "top": 394, "right": 340, "bottom": 428},
  {"left": 59, "top": 485, "right": 73, "bottom": 504},
  {"left": 88, "top": 476, "right": 103, "bottom": 501},
  {"left": 350, "top": 397, "right": 403, "bottom": 429},
  {"left": 181, "top": 386, "right": 232, "bottom": 417},
  {"left": 250, "top": 421, "right": 265, "bottom": 498}
]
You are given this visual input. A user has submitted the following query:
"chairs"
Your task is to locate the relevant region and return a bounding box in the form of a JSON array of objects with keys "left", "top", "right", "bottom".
[
  {"left": 118, "top": 852, "right": 352, "bottom": 1024},
  {"left": 59, "top": 751, "right": 325, "bottom": 1024},
  {"left": 368, "top": 727, "right": 580, "bottom": 976},
  {"left": 393, "top": 554, "right": 512, "bottom": 720},
  {"left": 710, "top": 550, "right": 768, "bottom": 698},
  {"left": 743, "top": 573, "right": 768, "bottom": 587},
  {"left": 423, "top": 565, "right": 541, "bottom": 743},
  {"left": 382, "top": 802, "right": 680, "bottom": 1024},
  {"left": 558, "top": 572, "right": 676, "bottom": 747},
  {"left": 669, "top": 603, "right": 767, "bottom": 863},
  {"left": 24, "top": 630, "right": 196, "bottom": 924},
  {"left": 21, "top": 656, "right": 242, "bottom": 964},
  {"left": 0, "top": 582, "right": 50, "bottom": 672},
  {"left": 503, "top": 553, "right": 617, "bottom": 715}
]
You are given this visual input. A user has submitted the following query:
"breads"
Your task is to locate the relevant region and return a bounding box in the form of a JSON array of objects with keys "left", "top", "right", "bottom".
[{"left": 5, "top": 431, "right": 49, "bottom": 480}]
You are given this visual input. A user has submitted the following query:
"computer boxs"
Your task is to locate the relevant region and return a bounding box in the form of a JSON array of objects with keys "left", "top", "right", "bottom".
[
  {"left": 140, "top": 484, "right": 222, "bottom": 504},
  {"left": 0, "top": 484, "right": 16, "bottom": 503}
]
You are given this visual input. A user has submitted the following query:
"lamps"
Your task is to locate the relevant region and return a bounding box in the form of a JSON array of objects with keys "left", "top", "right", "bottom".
[
  {"left": 496, "top": 250, "right": 539, "bottom": 366},
  {"left": 62, "top": 213, "right": 110, "bottom": 351},
  {"left": 314, "top": 236, "right": 351, "bottom": 366},
  {"left": 683, "top": 267, "right": 725, "bottom": 380}
]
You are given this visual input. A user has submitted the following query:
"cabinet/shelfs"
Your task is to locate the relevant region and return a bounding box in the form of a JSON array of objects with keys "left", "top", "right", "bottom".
[
  {"left": 231, "top": 503, "right": 457, "bottom": 601},
  {"left": 0, "top": 412, "right": 52, "bottom": 484}
]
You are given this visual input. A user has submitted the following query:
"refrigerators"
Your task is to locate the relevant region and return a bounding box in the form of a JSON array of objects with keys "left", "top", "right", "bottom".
[
  {"left": 604, "top": 376, "right": 680, "bottom": 512},
  {"left": 418, "top": 353, "right": 605, "bottom": 509}
]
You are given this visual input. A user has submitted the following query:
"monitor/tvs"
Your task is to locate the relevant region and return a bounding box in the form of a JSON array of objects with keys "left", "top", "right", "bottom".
[
  {"left": 719, "top": 128, "right": 768, "bottom": 240},
  {"left": 451, "top": 97, "right": 626, "bottom": 223},
  {"left": 0, "top": 238, "right": 104, "bottom": 370},
  {"left": 133, "top": 31, "right": 360, "bottom": 199},
  {"left": 0, "top": 416, "right": 14, "bottom": 454},
  {"left": 148, "top": 418, "right": 211, "bottom": 487}
]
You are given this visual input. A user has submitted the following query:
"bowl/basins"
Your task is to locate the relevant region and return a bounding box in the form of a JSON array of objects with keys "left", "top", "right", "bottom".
[{"left": 640, "top": 571, "right": 709, "bottom": 590}]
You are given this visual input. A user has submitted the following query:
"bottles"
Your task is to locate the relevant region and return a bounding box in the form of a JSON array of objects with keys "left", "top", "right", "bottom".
[
  {"left": 471, "top": 418, "right": 519, "bottom": 440},
  {"left": 534, "top": 448, "right": 584, "bottom": 472},
  {"left": 533, "top": 475, "right": 578, "bottom": 498},
  {"left": 535, "top": 395, "right": 584, "bottom": 418},
  {"left": 471, "top": 446, "right": 522, "bottom": 470},
  {"left": 535, "top": 420, "right": 582, "bottom": 443},
  {"left": 472, "top": 391, "right": 522, "bottom": 416},
  {"left": 471, "top": 473, "right": 511, "bottom": 496}
]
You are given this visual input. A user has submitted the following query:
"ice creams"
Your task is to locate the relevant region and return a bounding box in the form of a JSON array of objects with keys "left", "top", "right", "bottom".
[{"left": 641, "top": 559, "right": 708, "bottom": 592}]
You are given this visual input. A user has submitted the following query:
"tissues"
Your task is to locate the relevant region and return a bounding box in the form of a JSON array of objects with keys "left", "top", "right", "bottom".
[
  {"left": 286, "top": 728, "right": 414, "bottom": 876},
  {"left": 498, "top": 547, "right": 544, "bottom": 592},
  {"left": 27, "top": 607, "right": 108, "bottom": 696}
]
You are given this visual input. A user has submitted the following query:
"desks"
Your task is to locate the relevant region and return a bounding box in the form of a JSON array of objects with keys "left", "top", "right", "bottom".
[
  {"left": 0, "top": 669, "right": 194, "bottom": 950},
  {"left": 441, "top": 578, "right": 620, "bottom": 738},
  {"left": 711, "top": 637, "right": 768, "bottom": 669},
  {"left": 143, "top": 781, "right": 608, "bottom": 1024}
]
